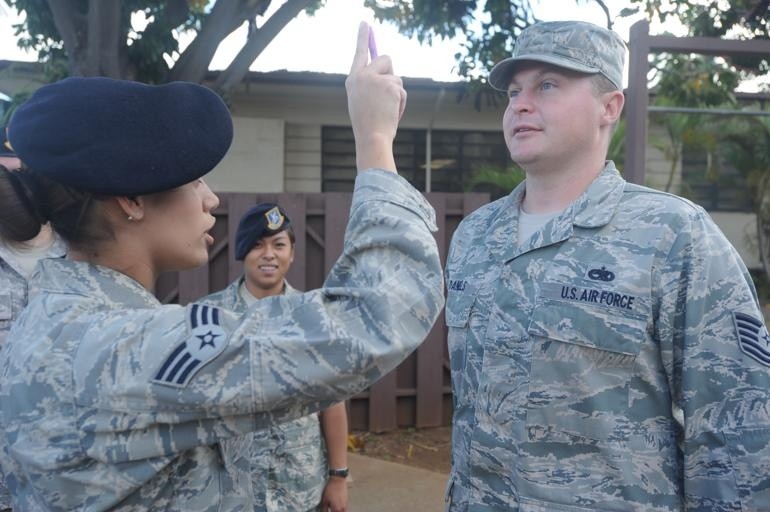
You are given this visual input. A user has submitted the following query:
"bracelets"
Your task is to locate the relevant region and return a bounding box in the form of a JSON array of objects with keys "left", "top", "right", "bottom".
[{"left": 328, "top": 465, "right": 348, "bottom": 479}]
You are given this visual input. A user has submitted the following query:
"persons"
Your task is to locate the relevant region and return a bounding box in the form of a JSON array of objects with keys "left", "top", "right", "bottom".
[
  {"left": 445, "top": 20, "right": 769, "bottom": 512},
  {"left": 2, "top": 21, "right": 446, "bottom": 511},
  {"left": 1, "top": 132, "right": 57, "bottom": 329},
  {"left": 176, "top": 199, "right": 350, "bottom": 511}
]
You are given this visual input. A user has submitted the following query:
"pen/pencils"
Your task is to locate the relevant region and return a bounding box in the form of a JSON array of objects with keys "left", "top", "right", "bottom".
[{"left": 369, "top": 25, "right": 378, "bottom": 63}]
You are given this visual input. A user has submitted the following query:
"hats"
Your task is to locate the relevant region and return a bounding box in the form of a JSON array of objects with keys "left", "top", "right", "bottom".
[
  {"left": 488, "top": 20, "right": 630, "bottom": 92},
  {"left": 0, "top": 124, "right": 19, "bottom": 158},
  {"left": 234, "top": 202, "right": 290, "bottom": 261},
  {"left": 8, "top": 77, "right": 233, "bottom": 197}
]
